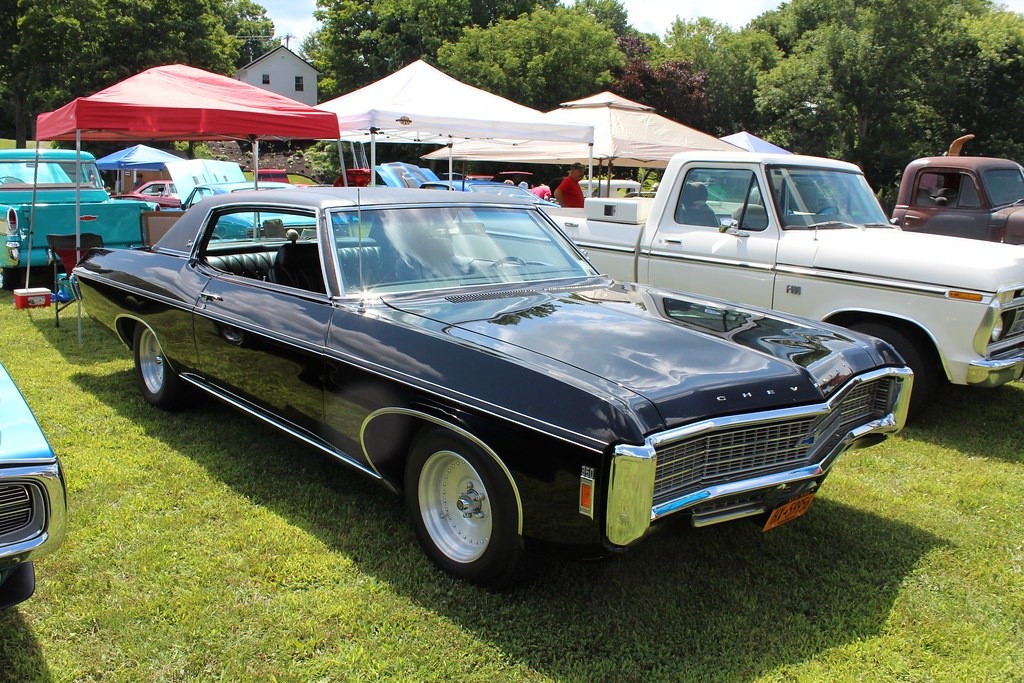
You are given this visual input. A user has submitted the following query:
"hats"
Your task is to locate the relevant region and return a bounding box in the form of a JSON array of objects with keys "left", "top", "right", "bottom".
[{"left": 569, "top": 162, "right": 584, "bottom": 169}]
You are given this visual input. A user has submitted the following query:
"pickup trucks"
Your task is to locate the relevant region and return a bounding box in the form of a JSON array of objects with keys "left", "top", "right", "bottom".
[
  {"left": 443, "top": 150, "right": 1024, "bottom": 433},
  {"left": 0, "top": 148, "right": 162, "bottom": 292},
  {"left": 159, "top": 180, "right": 360, "bottom": 241},
  {"left": 844, "top": 156, "right": 1024, "bottom": 247}
]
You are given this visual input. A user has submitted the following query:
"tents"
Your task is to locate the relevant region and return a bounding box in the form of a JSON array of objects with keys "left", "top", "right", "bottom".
[
  {"left": 94, "top": 143, "right": 187, "bottom": 184},
  {"left": 26, "top": 62, "right": 352, "bottom": 341},
  {"left": 419, "top": 90, "right": 752, "bottom": 198},
  {"left": 691, "top": 130, "right": 795, "bottom": 174},
  {"left": 311, "top": 59, "right": 594, "bottom": 198}
]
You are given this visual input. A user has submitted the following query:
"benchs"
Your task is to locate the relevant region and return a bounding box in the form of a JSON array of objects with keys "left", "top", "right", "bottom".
[{"left": 206, "top": 247, "right": 392, "bottom": 286}]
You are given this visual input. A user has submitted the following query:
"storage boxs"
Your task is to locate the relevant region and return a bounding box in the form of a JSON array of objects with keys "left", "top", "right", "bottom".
[{"left": 13, "top": 287, "right": 51, "bottom": 309}]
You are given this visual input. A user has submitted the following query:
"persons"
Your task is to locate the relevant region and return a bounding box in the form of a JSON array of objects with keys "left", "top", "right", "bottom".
[
  {"left": 650, "top": 183, "right": 659, "bottom": 197},
  {"left": 554, "top": 163, "right": 584, "bottom": 208},
  {"left": 131, "top": 173, "right": 146, "bottom": 192},
  {"left": 89, "top": 174, "right": 104, "bottom": 188},
  {"left": 106, "top": 186, "right": 116, "bottom": 196},
  {"left": 530, "top": 178, "right": 551, "bottom": 201},
  {"left": 503, "top": 180, "right": 515, "bottom": 186},
  {"left": 518, "top": 179, "right": 530, "bottom": 190}
]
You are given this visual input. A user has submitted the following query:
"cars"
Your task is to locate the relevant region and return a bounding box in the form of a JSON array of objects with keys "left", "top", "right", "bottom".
[
  {"left": 112, "top": 179, "right": 183, "bottom": 209},
  {"left": 255, "top": 162, "right": 562, "bottom": 246},
  {"left": 71, "top": 186, "right": 916, "bottom": 597}
]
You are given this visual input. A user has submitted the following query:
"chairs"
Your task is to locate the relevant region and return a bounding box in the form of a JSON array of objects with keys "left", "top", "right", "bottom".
[
  {"left": 682, "top": 182, "right": 720, "bottom": 226},
  {"left": 139, "top": 211, "right": 186, "bottom": 247},
  {"left": 272, "top": 243, "right": 326, "bottom": 294},
  {"left": 935, "top": 188, "right": 957, "bottom": 206},
  {"left": 747, "top": 187, "right": 781, "bottom": 228},
  {"left": 385, "top": 238, "right": 453, "bottom": 281},
  {"left": 45, "top": 232, "right": 105, "bottom": 328}
]
